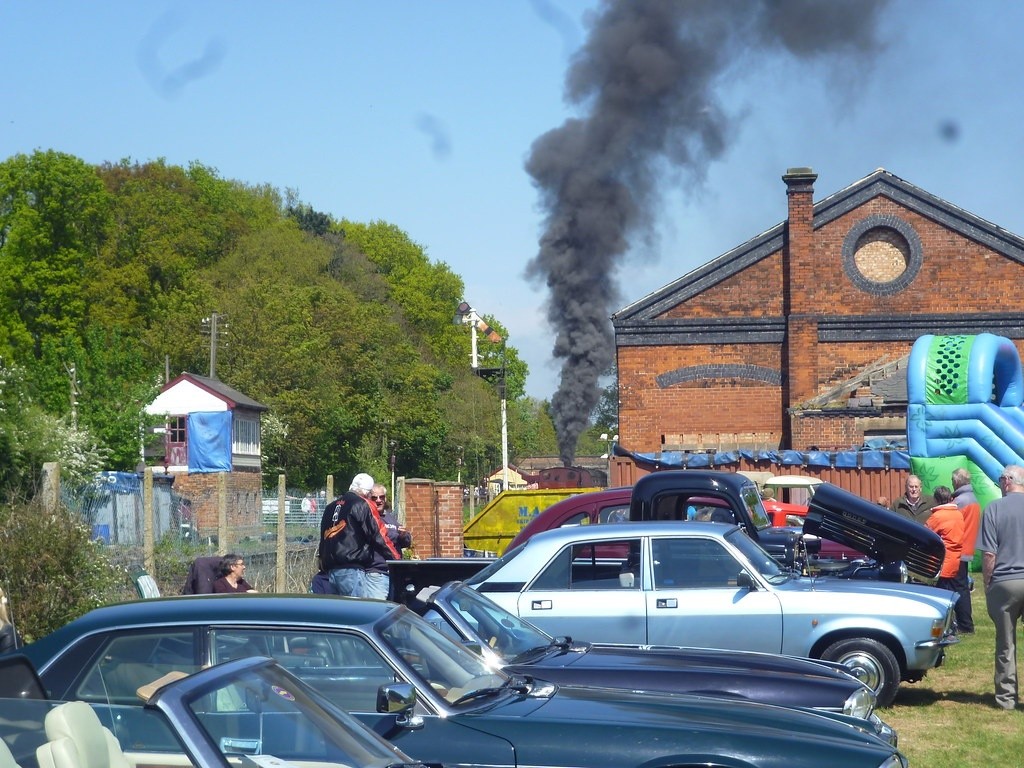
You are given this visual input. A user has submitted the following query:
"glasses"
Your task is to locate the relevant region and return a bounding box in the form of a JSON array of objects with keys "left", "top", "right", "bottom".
[
  {"left": 370, "top": 496, "right": 385, "bottom": 501},
  {"left": 230, "top": 563, "right": 244, "bottom": 567},
  {"left": 999, "top": 477, "right": 1013, "bottom": 482}
]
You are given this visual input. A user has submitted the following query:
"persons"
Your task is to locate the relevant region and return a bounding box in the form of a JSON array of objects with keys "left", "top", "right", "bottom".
[
  {"left": 975, "top": 464, "right": 1024, "bottom": 713},
  {"left": 302, "top": 473, "right": 414, "bottom": 600},
  {"left": 214, "top": 553, "right": 258, "bottom": 594},
  {"left": 951, "top": 468, "right": 983, "bottom": 633},
  {"left": 889, "top": 474, "right": 938, "bottom": 527},
  {"left": 924, "top": 486, "right": 974, "bottom": 635}
]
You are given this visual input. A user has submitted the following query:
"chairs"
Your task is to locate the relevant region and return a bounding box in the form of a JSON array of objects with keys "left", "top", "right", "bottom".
[
  {"left": 184, "top": 556, "right": 256, "bottom": 595},
  {"left": 125, "top": 566, "right": 162, "bottom": 600},
  {"left": 106, "top": 663, "right": 163, "bottom": 704},
  {"left": 36, "top": 700, "right": 137, "bottom": 767},
  {"left": 216, "top": 684, "right": 251, "bottom": 714}
]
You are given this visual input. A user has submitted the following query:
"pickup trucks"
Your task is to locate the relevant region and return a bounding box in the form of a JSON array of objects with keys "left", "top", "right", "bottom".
[{"left": 388, "top": 470, "right": 946, "bottom": 615}]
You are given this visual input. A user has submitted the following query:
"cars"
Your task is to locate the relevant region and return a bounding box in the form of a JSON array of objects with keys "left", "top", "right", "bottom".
[
  {"left": 0, "top": 579, "right": 908, "bottom": 768},
  {"left": 426, "top": 520, "right": 960, "bottom": 703},
  {"left": 503, "top": 488, "right": 865, "bottom": 560}
]
